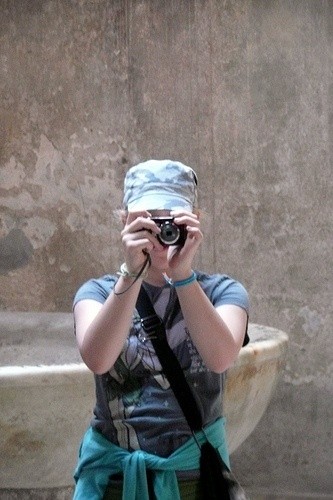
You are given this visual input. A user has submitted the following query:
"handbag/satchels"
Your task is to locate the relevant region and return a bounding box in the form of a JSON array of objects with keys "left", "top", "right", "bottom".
[{"left": 194, "top": 442, "right": 246, "bottom": 500}]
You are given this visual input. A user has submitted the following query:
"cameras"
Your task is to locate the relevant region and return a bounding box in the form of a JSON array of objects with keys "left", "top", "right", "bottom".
[{"left": 144, "top": 217, "right": 187, "bottom": 247}]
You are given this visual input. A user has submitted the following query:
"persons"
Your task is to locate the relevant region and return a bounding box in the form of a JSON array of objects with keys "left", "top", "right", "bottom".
[{"left": 72, "top": 160, "right": 249, "bottom": 500}]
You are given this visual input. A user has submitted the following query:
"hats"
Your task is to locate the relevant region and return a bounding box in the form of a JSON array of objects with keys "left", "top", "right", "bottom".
[{"left": 123, "top": 159, "right": 198, "bottom": 213}]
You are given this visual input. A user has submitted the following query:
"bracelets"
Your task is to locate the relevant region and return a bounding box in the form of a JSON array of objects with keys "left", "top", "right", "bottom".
[
  {"left": 117, "top": 263, "right": 148, "bottom": 278},
  {"left": 172, "top": 271, "right": 197, "bottom": 286}
]
